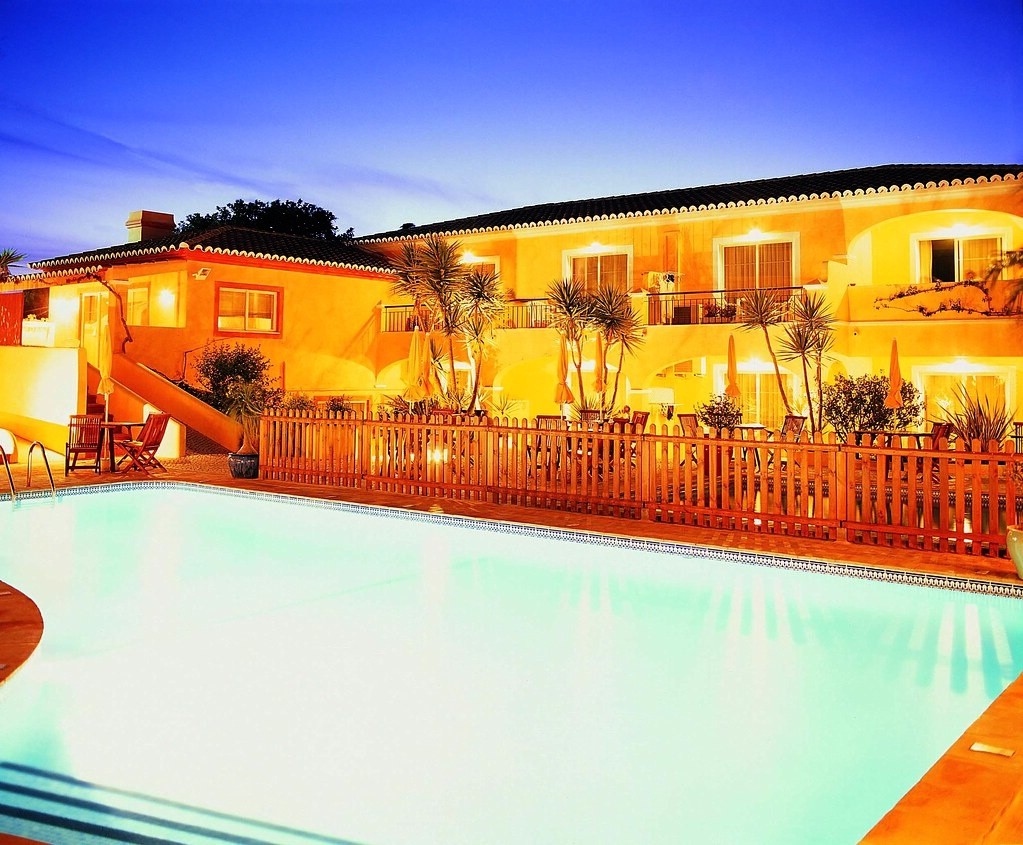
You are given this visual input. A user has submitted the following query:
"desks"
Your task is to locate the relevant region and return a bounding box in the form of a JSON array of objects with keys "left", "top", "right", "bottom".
[
  {"left": 68, "top": 421, "right": 147, "bottom": 471},
  {"left": 854, "top": 430, "right": 935, "bottom": 448},
  {"left": 734, "top": 424, "right": 767, "bottom": 467}
]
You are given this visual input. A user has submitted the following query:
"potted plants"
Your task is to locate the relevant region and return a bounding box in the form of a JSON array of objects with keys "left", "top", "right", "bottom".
[
  {"left": 224, "top": 375, "right": 286, "bottom": 480},
  {"left": 1003, "top": 458, "right": 1023, "bottom": 580}
]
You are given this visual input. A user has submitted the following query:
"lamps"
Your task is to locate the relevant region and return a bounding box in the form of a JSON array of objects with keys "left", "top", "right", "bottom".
[{"left": 193, "top": 268, "right": 211, "bottom": 281}]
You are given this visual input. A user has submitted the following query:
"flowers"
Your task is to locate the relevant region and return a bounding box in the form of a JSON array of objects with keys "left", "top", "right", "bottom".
[{"left": 704, "top": 303, "right": 720, "bottom": 314}]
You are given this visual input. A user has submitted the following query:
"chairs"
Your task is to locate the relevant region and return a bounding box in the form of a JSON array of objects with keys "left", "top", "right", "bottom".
[
  {"left": 527, "top": 410, "right": 650, "bottom": 482},
  {"left": 526, "top": 300, "right": 555, "bottom": 328},
  {"left": 677, "top": 413, "right": 701, "bottom": 467},
  {"left": 920, "top": 421, "right": 953, "bottom": 471},
  {"left": 65, "top": 411, "right": 172, "bottom": 475},
  {"left": 768, "top": 416, "right": 808, "bottom": 469},
  {"left": 379, "top": 407, "right": 491, "bottom": 476}
]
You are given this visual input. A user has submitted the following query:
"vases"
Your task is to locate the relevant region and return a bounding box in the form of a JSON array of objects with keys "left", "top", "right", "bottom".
[{"left": 707, "top": 313, "right": 716, "bottom": 317}]
